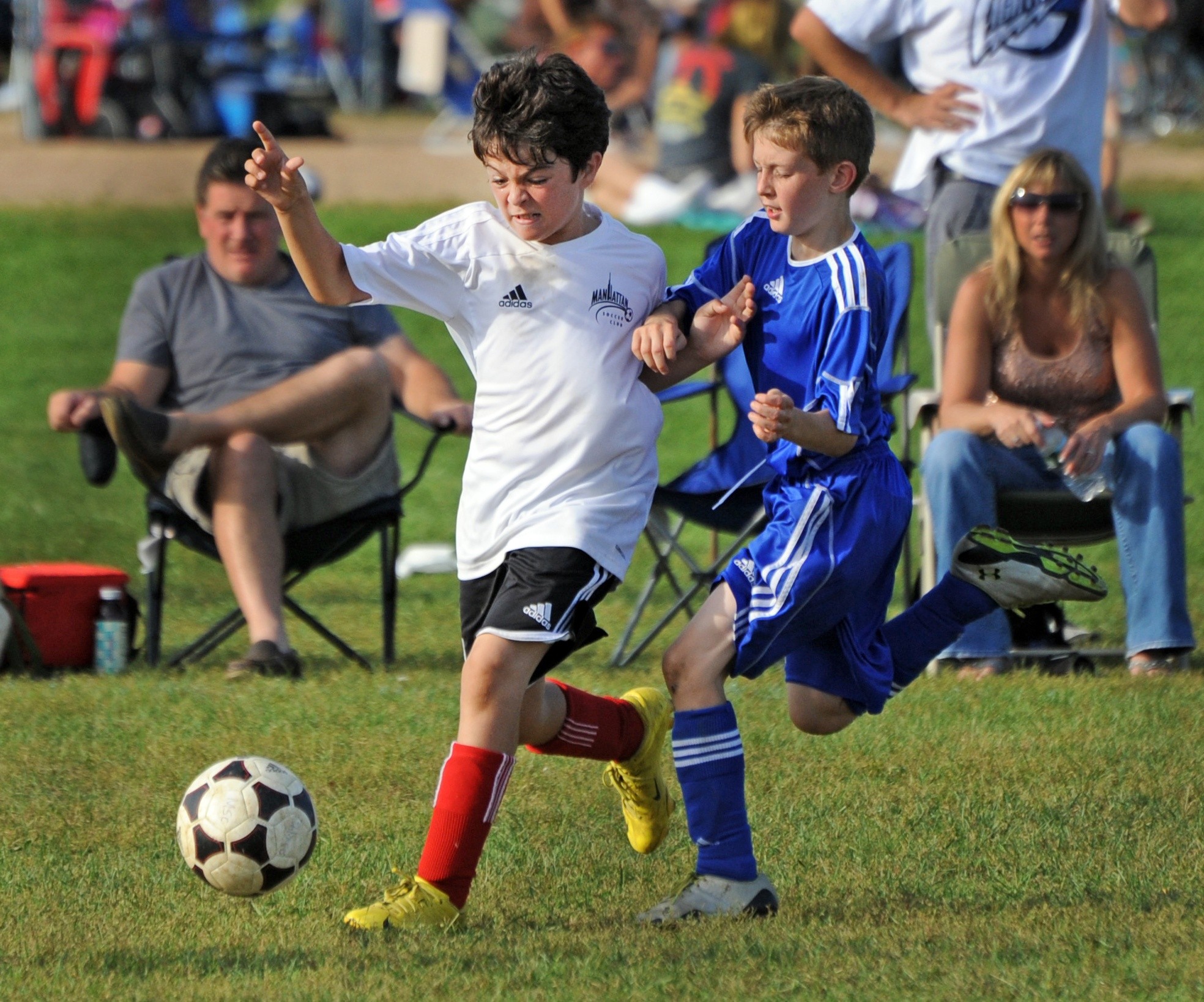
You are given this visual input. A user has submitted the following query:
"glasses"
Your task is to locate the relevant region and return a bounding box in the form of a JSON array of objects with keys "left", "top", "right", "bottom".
[{"left": 1009, "top": 192, "right": 1087, "bottom": 214}]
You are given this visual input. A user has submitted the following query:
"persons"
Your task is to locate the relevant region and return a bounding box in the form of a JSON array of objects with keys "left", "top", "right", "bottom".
[
  {"left": 631, "top": 77, "right": 1109, "bottom": 928},
  {"left": 244, "top": 47, "right": 756, "bottom": 931},
  {"left": 44, "top": 0, "right": 1204, "bottom": 688}
]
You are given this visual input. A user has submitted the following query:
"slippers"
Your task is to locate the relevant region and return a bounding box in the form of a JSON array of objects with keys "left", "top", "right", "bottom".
[
  {"left": 958, "top": 659, "right": 1004, "bottom": 677},
  {"left": 1128, "top": 655, "right": 1169, "bottom": 677}
]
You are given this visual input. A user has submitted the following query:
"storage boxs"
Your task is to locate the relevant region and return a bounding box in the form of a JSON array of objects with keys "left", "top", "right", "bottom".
[{"left": 0, "top": 562, "right": 131, "bottom": 668}]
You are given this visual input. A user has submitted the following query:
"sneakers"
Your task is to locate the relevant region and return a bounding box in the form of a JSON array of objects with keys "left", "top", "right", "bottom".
[
  {"left": 634, "top": 875, "right": 780, "bottom": 924},
  {"left": 223, "top": 640, "right": 304, "bottom": 680},
  {"left": 343, "top": 874, "right": 468, "bottom": 933},
  {"left": 950, "top": 523, "right": 1108, "bottom": 607},
  {"left": 601, "top": 687, "right": 675, "bottom": 853},
  {"left": 100, "top": 388, "right": 181, "bottom": 489}
]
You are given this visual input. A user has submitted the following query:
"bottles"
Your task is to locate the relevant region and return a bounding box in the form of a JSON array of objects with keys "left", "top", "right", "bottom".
[
  {"left": 94, "top": 586, "right": 130, "bottom": 678},
  {"left": 1033, "top": 421, "right": 1112, "bottom": 503}
]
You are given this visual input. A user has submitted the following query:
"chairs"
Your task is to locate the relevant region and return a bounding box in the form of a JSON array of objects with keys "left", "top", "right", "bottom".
[
  {"left": 906, "top": 230, "right": 1197, "bottom": 672},
  {"left": 605, "top": 240, "right": 920, "bottom": 671},
  {"left": 78, "top": 253, "right": 455, "bottom": 677}
]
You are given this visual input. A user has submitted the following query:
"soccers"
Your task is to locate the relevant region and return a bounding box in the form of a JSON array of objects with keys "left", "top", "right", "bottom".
[{"left": 174, "top": 756, "right": 321, "bottom": 900}]
